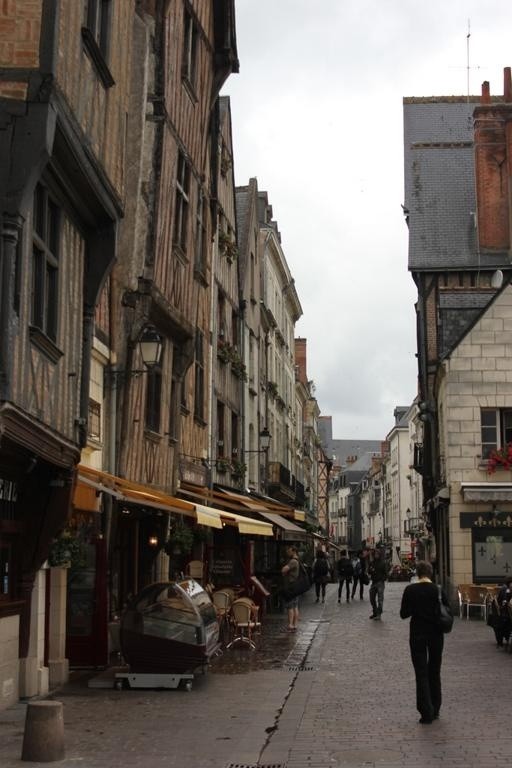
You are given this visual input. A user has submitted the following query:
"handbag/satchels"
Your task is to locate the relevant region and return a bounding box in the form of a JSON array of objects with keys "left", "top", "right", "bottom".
[
  {"left": 434, "top": 584, "right": 453, "bottom": 633},
  {"left": 363, "top": 574, "right": 370, "bottom": 586},
  {"left": 279, "top": 575, "right": 311, "bottom": 602}
]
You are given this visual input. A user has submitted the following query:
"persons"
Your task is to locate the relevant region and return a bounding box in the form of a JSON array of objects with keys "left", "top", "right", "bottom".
[
  {"left": 336, "top": 550, "right": 353, "bottom": 603},
  {"left": 352, "top": 555, "right": 366, "bottom": 600},
  {"left": 370, "top": 549, "right": 388, "bottom": 620},
  {"left": 311, "top": 549, "right": 330, "bottom": 603},
  {"left": 279, "top": 546, "right": 300, "bottom": 633},
  {"left": 401, "top": 561, "right": 451, "bottom": 724}
]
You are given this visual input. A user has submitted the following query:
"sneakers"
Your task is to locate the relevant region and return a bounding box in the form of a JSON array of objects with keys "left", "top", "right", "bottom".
[
  {"left": 279, "top": 624, "right": 296, "bottom": 632},
  {"left": 369, "top": 613, "right": 381, "bottom": 619}
]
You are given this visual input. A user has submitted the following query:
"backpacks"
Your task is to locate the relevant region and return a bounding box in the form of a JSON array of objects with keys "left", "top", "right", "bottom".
[
  {"left": 351, "top": 556, "right": 362, "bottom": 577},
  {"left": 314, "top": 558, "right": 328, "bottom": 576}
]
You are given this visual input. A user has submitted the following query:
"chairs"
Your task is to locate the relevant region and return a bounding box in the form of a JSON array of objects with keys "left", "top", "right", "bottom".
[
  {"left": 456, "top": 582, "right": 500, "bottom": 623},
  {"left": 208, "top": 584, "right": 262, "bottom": 652}
]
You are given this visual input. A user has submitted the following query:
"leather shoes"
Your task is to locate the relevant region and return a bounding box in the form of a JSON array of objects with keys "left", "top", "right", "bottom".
[{"left": 419, "top": 718, "right": 433, "bottom": 724}]
